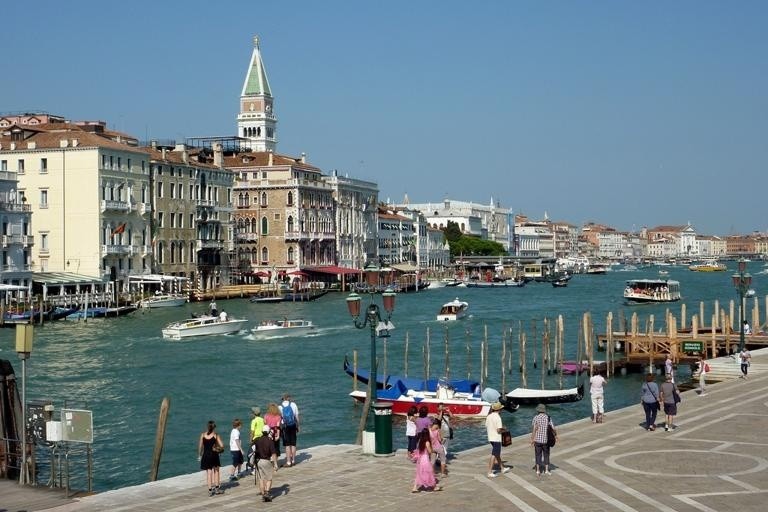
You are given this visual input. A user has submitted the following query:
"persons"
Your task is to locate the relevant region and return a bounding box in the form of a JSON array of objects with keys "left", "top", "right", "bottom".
[
  {"left": 665, "top": 351, "right": 676, "bottom": 383},
  {"left": 589, "top": 367, "right": 609, "bottom": 423},
  {"left": 404, "top": 402, "right": 453, "bottom": 493},
  {"left": 485, "top": 401, "right": 507, "bottom": 478},
  {"left": 228, "top": 393, "right": 300, "bottom": 502},
  {"left": 640, "top": 372, "right": 662, "bottom": 431},
  {"left": 659, "top": 372, "right": 681, "bottom": 432},
  {"left": 697, "top": 354, "right": 709, "bottom": 396},
  {"left": 743, "top": 320, "right": 750, "bottom": 335},
  {"left": 739, "top": 346, "right": 751, "bottom": 379},
  {"left": 529, "top": 404, "right": 556, "bottom": 478},
  {"left": 634, "top": 282, "right": 668, "bottom": 297},
  {"left": 219, "top": 308, "right": 229, "bottom": 322},
  {"left": 208, "top": 299, "right": 217, "bottom": 317},
  {"left": 197, "top": 420, "right": 225, "bottom": 497}
]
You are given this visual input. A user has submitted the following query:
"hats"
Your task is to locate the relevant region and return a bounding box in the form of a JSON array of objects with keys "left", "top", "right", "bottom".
[
  {"left": 252, "top": 407, "right": 261, "bottom": 414},
  {"left": 263, "top": 425, "right": 270, "bottom": 432},
  {"left": 281, "top": 394, "right": 290, "bottom": 400},
  {"left": 492, "top": 402, "right": 505, "bottom": 411},
  {"left": 536, "top": 404, "right": 545, "bottom": 413}
]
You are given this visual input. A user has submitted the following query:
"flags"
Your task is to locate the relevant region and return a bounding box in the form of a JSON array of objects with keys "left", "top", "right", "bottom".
[{"left": 111, "top": 224, "right": 125, "bottom": 239}]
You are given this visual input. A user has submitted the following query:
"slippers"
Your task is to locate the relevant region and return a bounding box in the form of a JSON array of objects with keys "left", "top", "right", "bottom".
[{"left": 281, "top": 460, "right": 295, "bottom": 467}]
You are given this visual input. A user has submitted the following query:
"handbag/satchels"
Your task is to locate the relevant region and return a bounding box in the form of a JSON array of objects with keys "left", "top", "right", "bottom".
[
  {"left": 592, "top": 413, "right": 604, "bottom": 424},
  {"left": 502, "top": 432, "right": 512, "bottom": 447},
  {"left": 672, "top": 389, "right": 681, "bottom": 404},
  {"left": 656, "top": 400, "right": 661, "bottom": 411},
  {"left": 705, "top": 364, "right": 709, "bottom": 372},
  {"left": 449, "top": 427, "right": 454, "bottom": 440},
  {"left": 547, "top": 424, "right": 556, "bottom": 447},
  {"left": 247, "top": 444, "right": 257, "bottom": 468},
  {"left": 409, "top": 450, "right": 418, "bottom": 462},
  {"left": 213, "top": 443, "right": 224, "bottom": 453}
]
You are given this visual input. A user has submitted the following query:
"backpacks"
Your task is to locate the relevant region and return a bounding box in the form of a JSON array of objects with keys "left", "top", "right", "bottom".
[{"left": 280, "top": 401, "right": 295, "bottom": 426}]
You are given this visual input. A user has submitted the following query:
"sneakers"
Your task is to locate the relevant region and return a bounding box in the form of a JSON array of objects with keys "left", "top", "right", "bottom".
[
  {"left": 273, "top": 465, "right": 279, "bottom": 470},
  {"left": 648, "top": 427, "right": 674, "bottom": 432},
  {"left": 209, "top": 490, "right": 224, "bottom": 496},
  {"left": 699, "top": 393, "right": 707, "bottom": 397},
  {"left": 536, "top": 471, "right": 551, "bottom": 477},
  {"left": 488, "top": 467, "right": 510, "bottom": 478},
  {"left": 250, "top": 472, "right": 255, "bottom": 475},
  {"left": 229, "top": 473, "right": 243, "bottom": 479},
  {"left": 263, "top": 494, "right": 272, "bottom": 502},
  {"left": 412, "top": 485, "right": 441, "bottom": 492},
  {"left": 441, "top": 474, "right": 447, "bottom": 478}
]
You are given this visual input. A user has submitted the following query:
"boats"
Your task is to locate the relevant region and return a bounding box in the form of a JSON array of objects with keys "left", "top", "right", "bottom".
[
  {"left": 247, "top": 263, "right": 463, "bottom": 302},
  {"left": 438, "top": 298, "right": 469, "bottom": 321},
  {"left": 160, "top": 317, "right": 318, "bottom": 340},
  {"left": 0, "top": 275, "right": 188, "bottom": 324},
  {"left": 345, "top": 354, "right": 640, "bottom": 420},
  {"left": 462, "top": 264, "right": 605, "bottom": 288},
  {"left": 660, "top": 270, "right": 668, "bottom": 275},
  {"left": 685, "top": 259, "right": 728, "bottom": 273},
  {"left": 623, "top": 279, "right": 682, "bottom": 303}
]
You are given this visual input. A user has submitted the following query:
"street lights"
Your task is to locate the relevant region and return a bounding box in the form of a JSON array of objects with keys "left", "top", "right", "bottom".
[
  {"left": 730, "top": 255, "right": 757, "bottom": 361},
  {"left": 342, "top": 283, "right": 396, "bottom": 432},
  {"left": 11, "top": 314, "right": 34, "bottom": 487}
]
